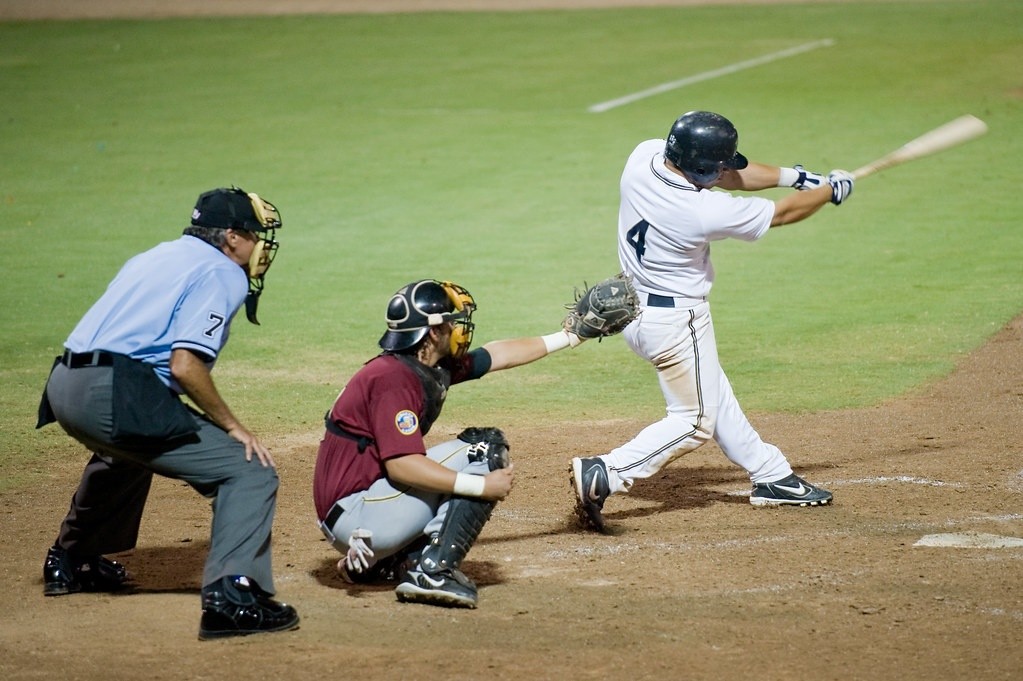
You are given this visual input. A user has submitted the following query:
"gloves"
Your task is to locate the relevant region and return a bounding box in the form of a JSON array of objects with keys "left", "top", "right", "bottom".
[
  {"left": 790, "top": 164, "right": 828, "bottom": 192},
  {"left": 828, "top": 169, "right": 855, "bottom": 206}
]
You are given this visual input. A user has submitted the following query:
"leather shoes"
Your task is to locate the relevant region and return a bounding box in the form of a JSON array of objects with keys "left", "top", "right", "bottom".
[
  {"left": 197, "top": 589, "right": 300, "bottom": 640},
  {"left": 42, "top": 545, "right": 126, "bottom": 595}
]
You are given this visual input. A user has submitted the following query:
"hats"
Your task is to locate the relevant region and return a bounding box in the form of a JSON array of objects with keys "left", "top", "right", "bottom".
[{"left": 190, "top": 187, "right": 268, "bottom": 234}]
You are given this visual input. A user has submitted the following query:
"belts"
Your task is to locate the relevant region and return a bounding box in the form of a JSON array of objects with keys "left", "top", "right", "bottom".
[
  {"left": 647, "top": 294, "right": 705, "bottom": 308},
  {"left": 60, "top": 347, "right": 114, "bottom": 369},
  {"left": 322, "top": 504, "right": 345, "bottom": 539}
]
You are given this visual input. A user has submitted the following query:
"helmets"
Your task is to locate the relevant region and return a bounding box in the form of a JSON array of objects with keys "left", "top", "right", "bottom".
[
  {"left": 378, "top": 279, "right": 455, "bottom": 351},
  {"left": 665, "top": 111, "right": 748, "bottom": 183}
]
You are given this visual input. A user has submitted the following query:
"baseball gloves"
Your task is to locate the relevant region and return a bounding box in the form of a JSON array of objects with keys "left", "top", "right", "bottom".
[{"left": 561, "top": 273, "right": 646, "bottom": 340}]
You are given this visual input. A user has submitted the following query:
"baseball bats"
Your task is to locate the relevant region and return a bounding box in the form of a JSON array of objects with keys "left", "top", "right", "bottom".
[{"left": 850, "top": 113, "right": 989, "bottom": 180}]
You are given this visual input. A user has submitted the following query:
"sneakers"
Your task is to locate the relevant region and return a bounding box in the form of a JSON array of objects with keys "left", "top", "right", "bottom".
[
  {"left": 748, "top": 472, "right": 833, "bottom": 507},
  {"left": 337, "top": 555, "right": 397, "bottom": 586},
  {"left": 394, "top": 565, "right": 478, "bottom": 609},
  {"left": 566, "top": 455, "right": 615, "bottom": 536}
]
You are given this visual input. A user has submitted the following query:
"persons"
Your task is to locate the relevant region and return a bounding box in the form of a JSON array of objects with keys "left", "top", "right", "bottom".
[
  {"left": 36, "top": 188, "right": 303, "bottom": 642},
  {"left": 564, "top": 109, "right": 856, "bottom": 534},
  {"left": 310, "top": 279, "right": 639, "bottom": 608}
]
